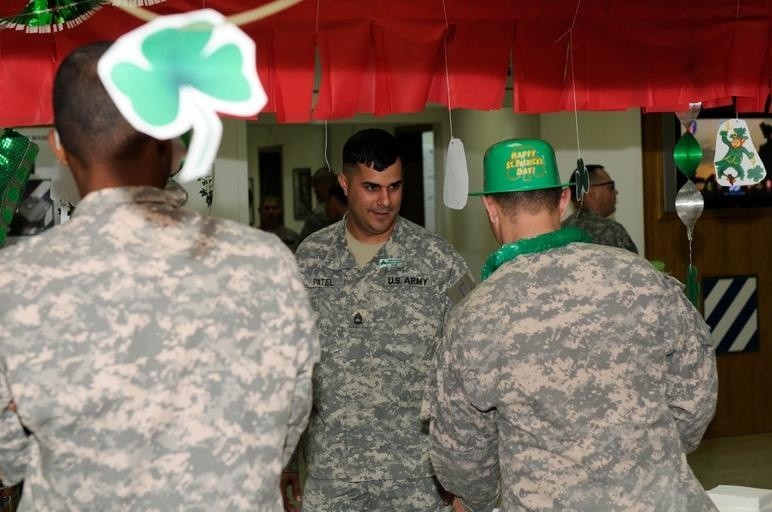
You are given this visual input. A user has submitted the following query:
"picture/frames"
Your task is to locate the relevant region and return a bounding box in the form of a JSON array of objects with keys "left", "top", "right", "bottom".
[{"left": 292, "top": 168, "right": 313, "bottom": 221}]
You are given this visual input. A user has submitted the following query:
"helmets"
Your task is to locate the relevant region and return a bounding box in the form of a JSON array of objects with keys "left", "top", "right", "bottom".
[{"left": 468, "top": 137, "right": 576, "bottom": 196}]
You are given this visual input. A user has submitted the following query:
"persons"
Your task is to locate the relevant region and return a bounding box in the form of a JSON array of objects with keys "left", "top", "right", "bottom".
[
  {"left": 425, "top": 137, "right": 720, "bottom": 510},
  {"left": 278, "top": 129, "right": 478, "bottom": 512},
  {"left": 561, "top": 165, "right": 640, "bottom": 255},
  {"left": 0, "top": 41, "right": 321, "bottom": 512},
  {"left": 255, "top": 166, "right": 346, "bottom": 254}
]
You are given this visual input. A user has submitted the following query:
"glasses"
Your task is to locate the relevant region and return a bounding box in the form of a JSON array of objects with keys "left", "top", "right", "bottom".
[{"left": 593, "top": 181, "right": 614, "bottom": 191}]
